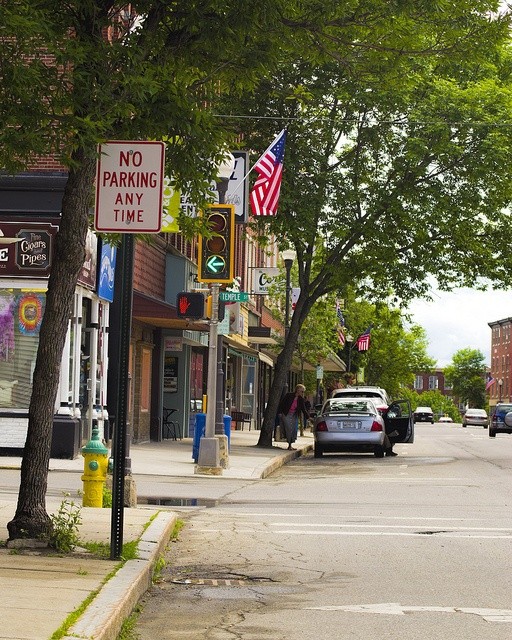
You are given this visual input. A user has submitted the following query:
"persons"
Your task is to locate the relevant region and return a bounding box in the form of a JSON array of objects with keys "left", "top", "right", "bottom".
[
  {"left": 384, "top": 437, "right": 398, "bottom": 456},
  {"left": 278, "top": 383, "right": 314, "bottom": 451},
  {"left": 303, "top": 395, "right": 312, "bottom": 431}
]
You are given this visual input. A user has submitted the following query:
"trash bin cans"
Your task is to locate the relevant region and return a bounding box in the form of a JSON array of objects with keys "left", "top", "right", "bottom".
[{"left": 192, "top": 414, "right": 232, "bottom": 464}]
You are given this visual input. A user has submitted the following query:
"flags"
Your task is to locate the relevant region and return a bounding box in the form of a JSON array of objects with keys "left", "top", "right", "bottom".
[
  {"left": 250, "top": 128, "right": 287, "bottom": 217},
  {"left": 333, "top": 301, "right": 346, "bottom": 345},
  {"left": 356, "top": 322, "right": 375, "bottom": 352},
  {"left": 484, "top": 373, "right": 497, "bottom": 391}
]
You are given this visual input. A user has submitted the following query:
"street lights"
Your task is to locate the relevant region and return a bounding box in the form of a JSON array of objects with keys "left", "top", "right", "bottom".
[
  {"left": 499, "top": 379, "right": 503, "bottom": 404},
  {"left": 281, "top": 250, "right": 296, "bottom": 393},
  {"left": 346, "top": 329, "right": 353, "bottom": 372}
]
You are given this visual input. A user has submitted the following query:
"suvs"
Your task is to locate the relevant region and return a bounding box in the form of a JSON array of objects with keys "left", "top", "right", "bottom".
[
  {"left": 489, "top": 404, "right": 512, "bottom": 437},
  {"left": 318, "top": 388, "right": 399, "bottom": 418}
]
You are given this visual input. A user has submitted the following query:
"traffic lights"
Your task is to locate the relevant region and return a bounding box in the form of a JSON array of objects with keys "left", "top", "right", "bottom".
[
  {"left": 197, "top": 204, "right": 235, "bottom": 284},
  {"left": 177, "top": 292, "right": 205, "bottom": 320}
]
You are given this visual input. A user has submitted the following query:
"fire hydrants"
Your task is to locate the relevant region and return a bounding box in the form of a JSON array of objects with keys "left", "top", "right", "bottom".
[{"left": 80, "top": 426, "right": 114, "bottom": 508}]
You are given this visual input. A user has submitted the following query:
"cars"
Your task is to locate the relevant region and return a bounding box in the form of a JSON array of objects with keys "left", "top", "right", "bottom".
[
  {"left": 309, "top": 398, "right": 414, "bottom": 457},
  {"left": 463, "top": 409, "right": 489, "bottom": 428},
  {"left": 413, "top": 406, "right": 434, "bottom": 424},
  {"left": 438, "top": 417, "right": 453, "bottom": 422}
]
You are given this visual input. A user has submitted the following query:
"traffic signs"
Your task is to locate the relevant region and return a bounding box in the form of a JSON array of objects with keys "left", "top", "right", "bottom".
[{"left": 219, "top": 292, "right": 248, "bottom": 302}]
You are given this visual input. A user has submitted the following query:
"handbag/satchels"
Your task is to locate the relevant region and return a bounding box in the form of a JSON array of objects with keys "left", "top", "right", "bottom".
[{"left": 275, "top": 424, "right": 286, "bottom": 442}]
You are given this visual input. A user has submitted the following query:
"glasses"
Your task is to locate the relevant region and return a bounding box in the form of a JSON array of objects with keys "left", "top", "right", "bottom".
[{"left": 301, "top": 391, "right": 304, "bottom": 393}]
